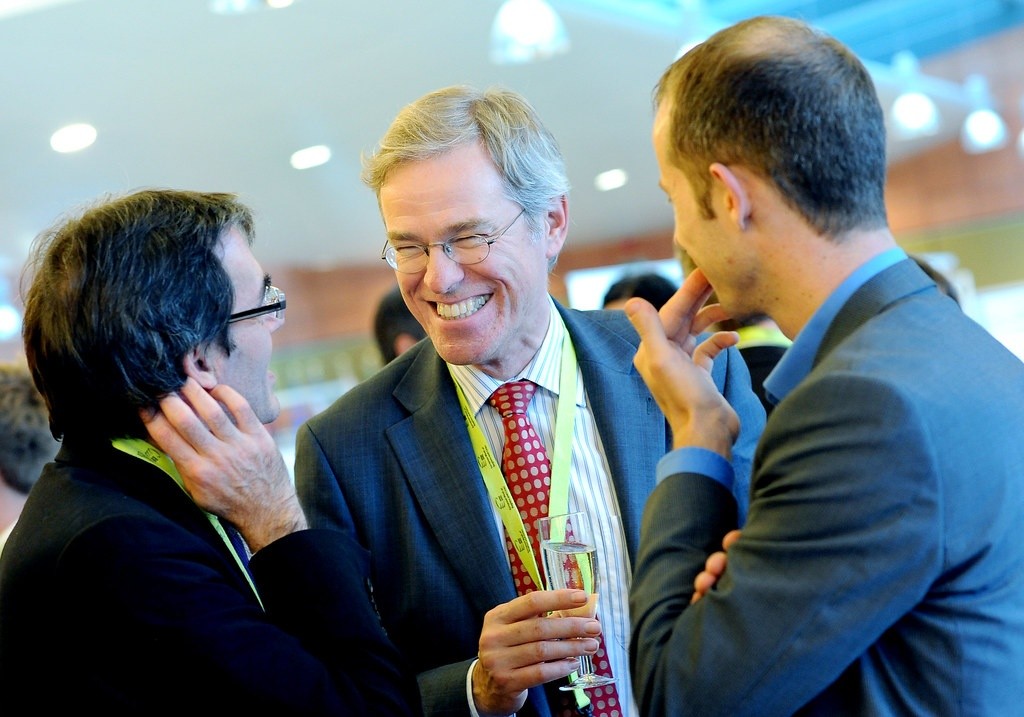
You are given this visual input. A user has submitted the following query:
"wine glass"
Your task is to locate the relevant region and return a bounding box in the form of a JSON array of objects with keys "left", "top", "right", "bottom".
[{"left": 537, "top": 509, "right": 620, "bottom": 691}]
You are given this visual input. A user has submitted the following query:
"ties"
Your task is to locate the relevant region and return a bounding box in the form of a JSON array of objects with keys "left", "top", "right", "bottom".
[{"left": 485, "top": 379, "right": 623, "bottom": 717}]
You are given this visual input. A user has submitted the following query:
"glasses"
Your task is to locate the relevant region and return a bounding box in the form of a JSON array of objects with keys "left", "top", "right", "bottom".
[
  {"left": 380, "top": 208, "right": 527, "bottom": 274},
  {"left": 227, "top": 285, "right": 287, "bottom": 323}
]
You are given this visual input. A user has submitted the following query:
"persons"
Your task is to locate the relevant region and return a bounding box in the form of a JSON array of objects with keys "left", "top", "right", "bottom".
[
  {"left": 0, "top": 180, "right": 424, "bottom": 717},
  {"left": 294, "top": 83, "right": 768, "bottom": 717},
  {"left": 0, "top": 357, "right": 62, "bottom": 559},
  {"left": 371, "top": 12, "right": 1023, "bottom": 717}
]
